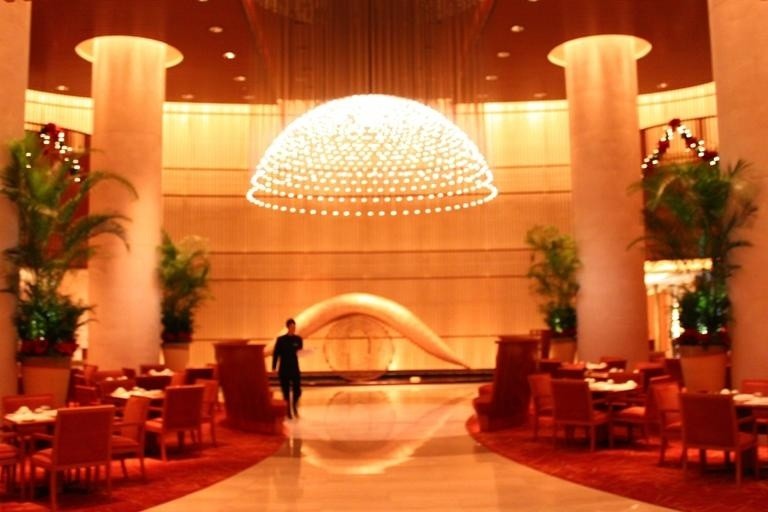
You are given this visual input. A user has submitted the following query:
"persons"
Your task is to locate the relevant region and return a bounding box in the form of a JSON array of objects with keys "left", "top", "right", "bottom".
[{"left": 270, "top": 317, "right": 307, "bottom": 423}]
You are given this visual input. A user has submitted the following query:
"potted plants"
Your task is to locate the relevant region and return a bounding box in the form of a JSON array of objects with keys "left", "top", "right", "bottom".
[
  {"left": 623, "top": 157, "right": 765, "bottom": 395},
  {"left": 1, "top": 122, "right": 140, "bottom": 406},
  {"left": 154, "top": 228, "right": 216, "bottom": 372},
  {"left": 519, "top": 218, "right": 583, "bottom": 365}
]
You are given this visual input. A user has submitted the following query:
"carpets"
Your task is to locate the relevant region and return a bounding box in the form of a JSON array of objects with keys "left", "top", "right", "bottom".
[{"left": 465, "top": 410, "right": 767, "bottom": 511}]
